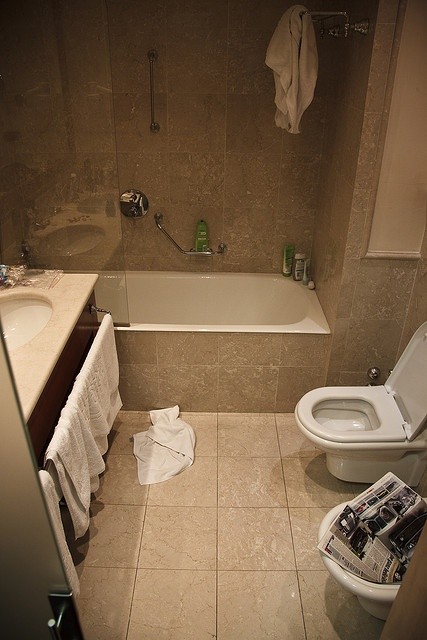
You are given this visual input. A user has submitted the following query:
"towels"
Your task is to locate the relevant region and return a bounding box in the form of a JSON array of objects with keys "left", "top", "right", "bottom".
[
  {"left": 266, "top": 5, "right": 318, "bottom": 135},
  {"left": 45, "top": 313, "right": 124, "bottom": 539},
  {"left": 132, "top": 404, "right": 196, "bottom": 486},
  {"left": 38, "top": 469, "right": 82, "bottom": 598}
]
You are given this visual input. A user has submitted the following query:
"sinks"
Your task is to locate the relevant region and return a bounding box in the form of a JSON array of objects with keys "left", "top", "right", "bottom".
[
  {"left": 32, "top": 224, "right": 106, "bottom": 257},
  {"left": 75, "top": 194, "right": 119, "bottom": 216},
  {"left": 0, "top": 290, "right": 55, "bottom": 354}
]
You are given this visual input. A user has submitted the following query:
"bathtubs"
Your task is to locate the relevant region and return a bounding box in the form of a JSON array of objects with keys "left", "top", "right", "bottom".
[
  {"left": 93, "top": 271, "right": 331, "bottom": 334},
  {"left": 318, "top": 498, "right": 427, "bottom": 621}
]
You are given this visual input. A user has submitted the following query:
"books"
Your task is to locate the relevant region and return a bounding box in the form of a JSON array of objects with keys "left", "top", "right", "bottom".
[{"left": 315, "top": 472, "right": 427, "bottom": 586}]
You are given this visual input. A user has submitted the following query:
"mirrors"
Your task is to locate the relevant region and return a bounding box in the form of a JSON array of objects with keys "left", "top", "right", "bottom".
[{"left": 118, "top": 189, "right": 149, "bottom": 219}]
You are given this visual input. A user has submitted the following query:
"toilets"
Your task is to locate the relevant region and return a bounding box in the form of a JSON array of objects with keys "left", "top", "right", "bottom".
[{"left": 295, "top": 321, "right": 426, "bottom": 487}]
